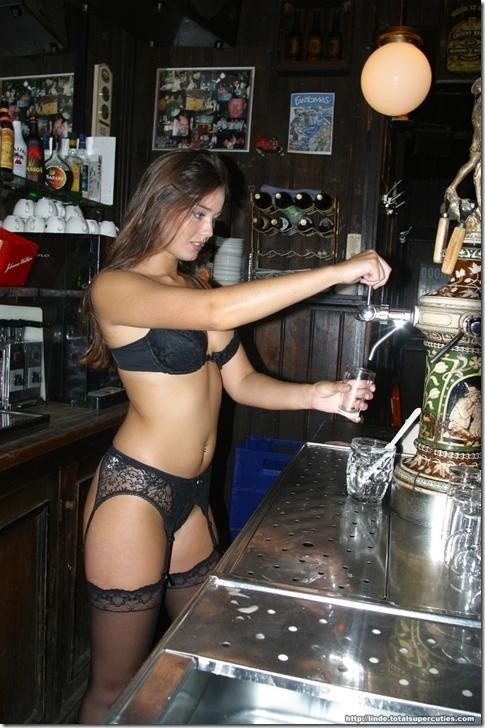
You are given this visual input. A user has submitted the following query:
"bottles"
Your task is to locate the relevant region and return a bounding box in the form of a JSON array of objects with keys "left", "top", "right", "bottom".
[
  {"left": 1, "top": 98, "right": 90, "bottom": 198},
  {"left": 248, "top": 186, "right": 338, "bottom": 238}
]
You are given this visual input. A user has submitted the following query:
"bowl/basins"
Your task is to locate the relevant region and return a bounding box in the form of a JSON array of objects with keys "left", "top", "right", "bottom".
[{"left": 213, "top": 234, "right": 244, "bottom": 286}]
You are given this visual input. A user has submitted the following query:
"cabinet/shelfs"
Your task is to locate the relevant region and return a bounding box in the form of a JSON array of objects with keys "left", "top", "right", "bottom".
[
  {"left": 246, "top": 193, "right": 341, "bottom": 281},
  {"left": 1, "top": 402, "right": 130, "bottom": 725}
]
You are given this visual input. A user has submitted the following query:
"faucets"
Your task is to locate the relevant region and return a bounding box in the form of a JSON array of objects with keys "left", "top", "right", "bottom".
[{"left": 353, "top": 284, "right": 416, "bottom": 362}]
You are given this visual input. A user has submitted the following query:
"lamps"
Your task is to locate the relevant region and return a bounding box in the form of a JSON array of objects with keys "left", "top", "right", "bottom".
[{"left": 359, "top": 0, "right": 432, "bottom": 118}]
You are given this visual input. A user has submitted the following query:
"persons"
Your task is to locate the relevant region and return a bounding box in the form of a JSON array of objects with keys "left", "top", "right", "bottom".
[
  {"left": 209, "top": 97, "right": 246, "bottom": 148},
  {"left": 66, "top": 142, "right": 393, "bottom": 725},
  {"left": 449, "top": 385, "right": 481, "bottom": 437}
]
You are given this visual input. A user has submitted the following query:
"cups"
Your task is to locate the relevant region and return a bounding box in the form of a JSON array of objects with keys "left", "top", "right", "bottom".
[
  {"left": 5, "top": 197, "right": 118, "bottom": 237},
  {"left": 339, "top": 365, "right": 377, "bottom": 415},
  {"left": 345, "top": 437, "right": 398, "bottom": 503},
  {"left": 444, "top": 464, "right": 482, "bottom": 593}
]
